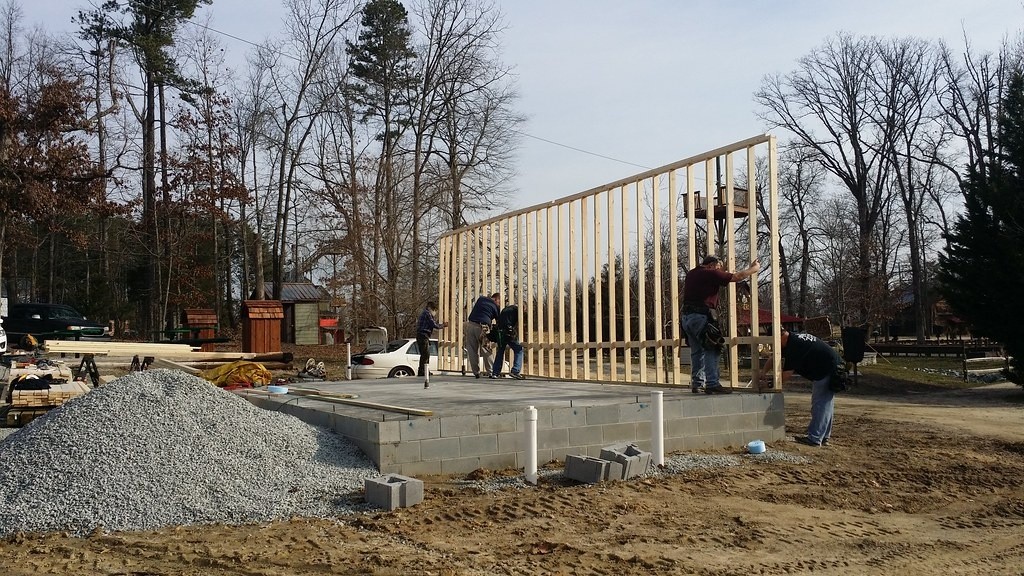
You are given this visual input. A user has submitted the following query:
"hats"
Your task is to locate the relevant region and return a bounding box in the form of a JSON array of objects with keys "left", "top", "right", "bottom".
[
  {"left": 767, "top": 325, "right": 784, "bottom": 338},
  {"left": 428, "top": 302, "right": 439, "bottom": 310}
]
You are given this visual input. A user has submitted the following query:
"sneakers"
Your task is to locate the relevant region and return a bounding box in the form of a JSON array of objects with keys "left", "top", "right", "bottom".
[
  {"left": 492, "top": 373, "right": 503, "bottom": 378},
  {"left": 510, "top": 371, "right": 525, "bottom": 380},
  {"left": 692, "top": 386, "right": 705, "bottom": 392},
  {"left": 704, "top": 386, "right": 732, "bottom": 394}
]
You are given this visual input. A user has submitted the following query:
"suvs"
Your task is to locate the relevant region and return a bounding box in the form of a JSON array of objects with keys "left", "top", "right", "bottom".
[{"left": 1, "top": 303, "right": 111, "bottom": 351}]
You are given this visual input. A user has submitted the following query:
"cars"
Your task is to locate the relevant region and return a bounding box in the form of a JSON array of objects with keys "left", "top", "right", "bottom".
[
  {"left": 343, "top": 326, "right": 512, "bottom": 379},
  {"left": 0, "top": 319, "right": 7, "bottom": 356}
]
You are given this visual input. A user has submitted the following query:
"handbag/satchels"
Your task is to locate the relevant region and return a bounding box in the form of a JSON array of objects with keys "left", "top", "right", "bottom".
[
  {"left": 831, "top": 360, "right": 849, "bottom": 391},
  {"left": 701, "top": 321, "right": 724, "bottom": 353}
]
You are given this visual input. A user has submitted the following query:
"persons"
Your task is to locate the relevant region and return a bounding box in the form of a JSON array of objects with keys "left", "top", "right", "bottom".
[
  {"left": 467, "top": 293, "right": 500, "bottom": 378},
  {"left": 760, "top": 325, "right": 846, "bottom": 446},
  {"left": 682, "top": 255, "right": 760, "bottom": 394},
  {"left": 417, "top": 303, "right": 449, "bottom": 376},
  {"left": 492, "top": 302, "right": 527, "bottom": 379}
]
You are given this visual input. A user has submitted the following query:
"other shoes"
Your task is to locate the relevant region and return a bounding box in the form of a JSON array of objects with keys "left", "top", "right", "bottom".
[
  {"left": 823, "top": 438, "right": 830, "bottom": 446},
  {"left": 418, "top": 371, "right": 433, "bottom": 376},
  {"left": 475, "top": 373, "right": 483, "bottom": 378},
  {"left": 794, "top": 436, "right": 820, "bottom": 447},
  {"left": 489, "top": 374, "right": 492, "bottom": 378}
]
